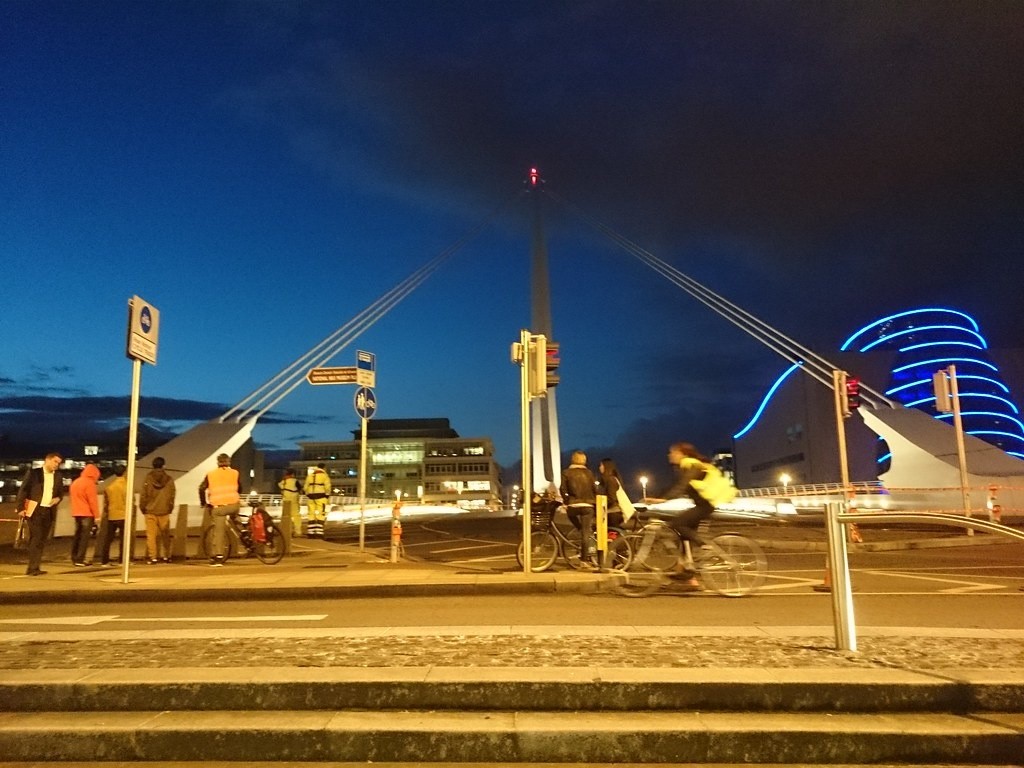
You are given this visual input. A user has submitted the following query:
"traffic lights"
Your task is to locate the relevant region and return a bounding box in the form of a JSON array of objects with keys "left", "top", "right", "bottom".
[
  {"left": 841, "top": 372, "right": 863, "bottom": 416},
  {"left": 528, "top": 333, "right": 564, "bottom": 399}
]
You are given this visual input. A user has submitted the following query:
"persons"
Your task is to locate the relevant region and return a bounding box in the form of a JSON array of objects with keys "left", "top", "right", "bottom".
[
  {"left": 139, "top": 456, "right": 176, "bottom": 565},
  {"left": 101, "top": 464, "right": 137, "bottom": 567},
  {"left": 102, "top": 461, "right": 120, "bottom": 478},
  {"left": 639, "top": 441, "right": 739, "bottom": 593},
  {"left": 560, "top": 450, "right": 604, "bottom": 564},
  {"left": 198, "top": 453, "right": 249, "bottom": 566},
  {"left": 277, "top": 467, "right": 305, "bottom": 537},
  {"left": 16, "top": 452, "right": 66, "bottom": 578},
  {"left": 301, "top": 462, "right": 332, "bottom": 541},
  {"left": 68, "top": 464, "right": 101, "bottom": 567},
  {"left": 593, "top": 458, "right": 635, "bottom": 570}
]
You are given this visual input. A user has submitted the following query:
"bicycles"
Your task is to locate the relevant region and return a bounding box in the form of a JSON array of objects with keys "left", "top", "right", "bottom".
[
  {"left": 614, "top": 505, "right": 768, "bottom": 606},
  {"left": 515, "top": 492, "right": 682, "bottom": 574},
  {"left": 201, "top": 499, "right": 287, "bottom": 565}
]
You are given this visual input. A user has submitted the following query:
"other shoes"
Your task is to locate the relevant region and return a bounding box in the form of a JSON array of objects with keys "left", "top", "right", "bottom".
[
  {"left": 292, "top": 533, "right": 304, "bottom": 537},
  {"left": 26, "top": 570, "right": 47, "bottom": 576},
  {"left": 163, "top": 557, "right": 173, "bottom": 563},
  {"left": 74, "top": 562, "right": 90, "bottom": 566},
  {"left": 668, "top": 569, "right": 701, "bottom": 586},
  {"left": 306, "top": 533, "right": 323, "bottom": 538},
  {"left": 146, "top": 558, "right": 158, "bottom": 564},
  {"left": 209, "top": 556, "right": 223, "bottom": 566},
  {"left": 580, "top": 561, "right": 594, "bottom": 569},
  {"left": 102, "top": 563, "right": 112, "bottom": 567}
]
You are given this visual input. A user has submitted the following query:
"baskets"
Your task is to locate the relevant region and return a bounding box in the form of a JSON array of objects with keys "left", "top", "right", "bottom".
[{"left": 530, "top": 511, "right": 551, "bottom": 526}]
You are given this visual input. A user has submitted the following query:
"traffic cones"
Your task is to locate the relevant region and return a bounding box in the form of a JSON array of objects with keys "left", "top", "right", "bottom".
[{"left": 813, "top": 547, "right": 835, "bottom": 593}]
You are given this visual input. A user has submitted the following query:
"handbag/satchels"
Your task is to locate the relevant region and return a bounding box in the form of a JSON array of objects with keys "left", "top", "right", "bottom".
[
  {"left": 611, "top": 475, "right": 636, "bottom": 524},
  {"left": 14, "top": 516, "right": 31, "bottom": 550}
]
